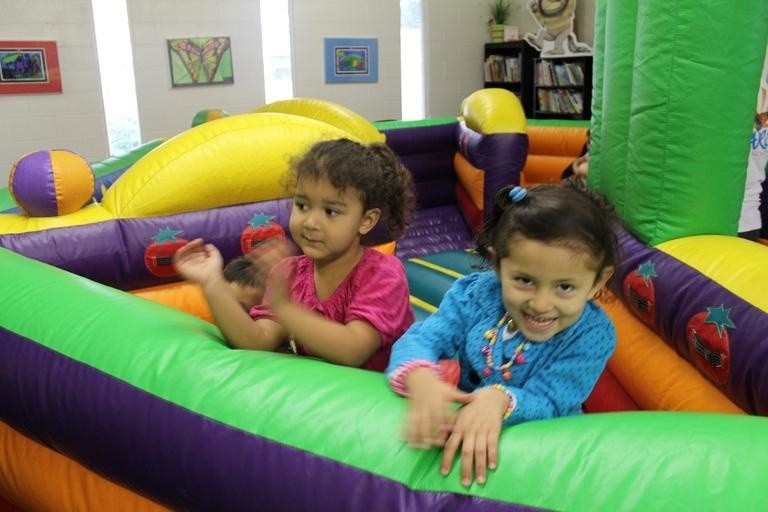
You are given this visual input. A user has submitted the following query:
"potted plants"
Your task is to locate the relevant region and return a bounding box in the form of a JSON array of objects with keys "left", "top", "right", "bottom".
[{"left": 486, "top": 0, "right": 517, "bottom": 41}]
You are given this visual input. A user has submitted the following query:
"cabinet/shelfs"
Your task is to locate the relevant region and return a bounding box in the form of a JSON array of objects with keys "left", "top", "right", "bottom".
[{"left": 481, "top": 40, "right": 593, "bottom": 119}]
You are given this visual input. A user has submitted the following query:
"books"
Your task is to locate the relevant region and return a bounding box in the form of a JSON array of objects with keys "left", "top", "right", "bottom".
[{"left": 483, "top": 52, "right": 586, "bottom": 114}]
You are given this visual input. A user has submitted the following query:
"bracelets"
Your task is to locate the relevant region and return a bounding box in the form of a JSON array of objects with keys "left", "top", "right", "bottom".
[
  {"left": 492, "top": 384, "right": 515, "bottom": 421},
  {"left": 389, "top": 359, "right": 445, "bottom": 398}
]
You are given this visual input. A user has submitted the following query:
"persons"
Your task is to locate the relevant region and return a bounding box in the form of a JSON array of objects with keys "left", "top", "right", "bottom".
[
  {"left": 222, "top": 254, "right": 297, "bottom": 355},
  {"left": 174, "top": 135, "right": 419, "bottom": 370},
  {"left": 386, "top": 180, "right": 618, "bottom": 488}
]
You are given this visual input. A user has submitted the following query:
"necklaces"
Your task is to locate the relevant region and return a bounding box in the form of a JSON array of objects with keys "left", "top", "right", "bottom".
[{"left": 478, "top": 312, "right": 529, "bottom": 383}]
